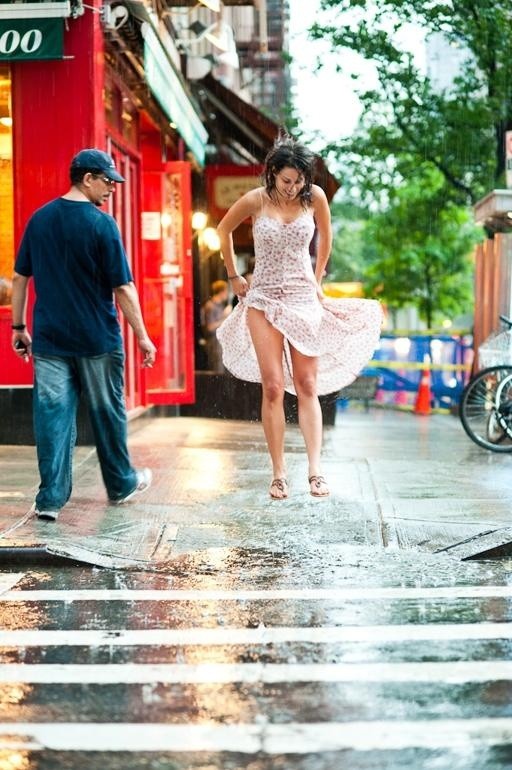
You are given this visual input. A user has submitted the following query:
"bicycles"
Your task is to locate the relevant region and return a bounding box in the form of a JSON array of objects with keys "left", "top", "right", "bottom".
[{"left": 458, "top": 314, "right": 512, "bottom": 453}]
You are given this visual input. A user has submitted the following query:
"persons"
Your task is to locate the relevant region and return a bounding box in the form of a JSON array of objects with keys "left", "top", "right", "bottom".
[
  {"left": 215, "top": 138, "right": 335, "bottom": 499},
  {"left": 197, "top": 280, "right": 234, "bottom": 370},
  {"left": 11, "top": 148, "right": 158, "bottom": 522}
]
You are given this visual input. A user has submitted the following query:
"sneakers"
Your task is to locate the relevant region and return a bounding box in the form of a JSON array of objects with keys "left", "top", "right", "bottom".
[
  {"left": 35, "top": 503, "right": 61, "bottom": 522},
  {"left": 109, "top": 467, "right": 153, "bottom": 504}
]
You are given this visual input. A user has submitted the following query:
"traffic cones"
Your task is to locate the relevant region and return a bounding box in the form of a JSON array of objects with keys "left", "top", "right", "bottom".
[{"left": 413, "top": 370, "right": 432, "bottom": 416}]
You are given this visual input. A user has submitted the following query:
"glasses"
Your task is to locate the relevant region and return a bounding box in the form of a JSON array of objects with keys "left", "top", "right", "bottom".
[{"left": 90, "top": 173, "right": 115, "bottom": 184}]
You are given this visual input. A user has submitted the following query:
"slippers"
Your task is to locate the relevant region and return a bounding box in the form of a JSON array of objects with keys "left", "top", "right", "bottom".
[
  {"left": 308, "top": 474, "right": 330, "bottom": 498},
  {"left": 269, "top": 477, "right": 290, "bottom": 501}
]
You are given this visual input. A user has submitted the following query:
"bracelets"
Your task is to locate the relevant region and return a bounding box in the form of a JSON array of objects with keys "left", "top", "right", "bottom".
[
  {"left": 227, "top": 275, "right": 240, "bottom": 281},
  {"left": 12, "top": 323, "right": 26, "bottom": 331}
]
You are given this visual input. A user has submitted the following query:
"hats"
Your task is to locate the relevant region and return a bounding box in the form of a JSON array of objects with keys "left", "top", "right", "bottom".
[{"left": 70, "top": 148, "right": 126, "bottom": 184}]
